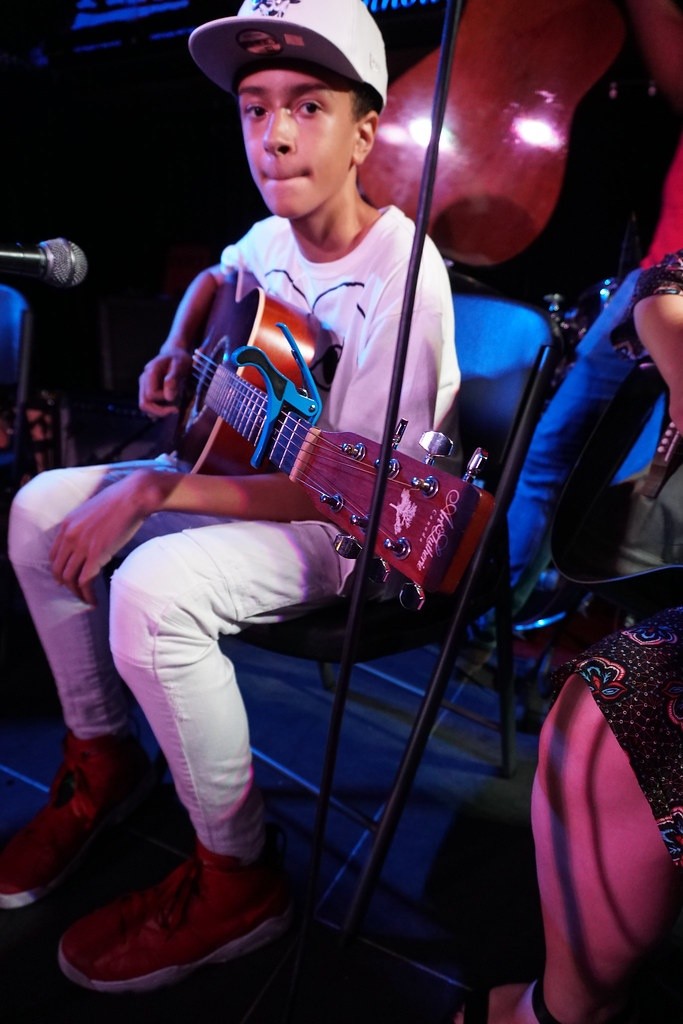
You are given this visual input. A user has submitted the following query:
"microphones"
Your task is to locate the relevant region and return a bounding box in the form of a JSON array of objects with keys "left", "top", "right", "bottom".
[{"left": 0, "top": 238, "right": 87, "bottom": 287}]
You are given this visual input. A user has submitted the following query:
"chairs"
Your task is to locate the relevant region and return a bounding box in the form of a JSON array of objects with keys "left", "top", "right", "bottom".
[{"left": 201, "top": 280, "right": 556, "bottom": 955}]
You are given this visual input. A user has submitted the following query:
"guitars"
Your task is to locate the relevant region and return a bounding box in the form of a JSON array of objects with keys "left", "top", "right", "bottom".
[
  {"left": 159, "top": 259, "right": 500, "bottom": 620},
  {"left": 547, "top": 349, "right": 683, "bottom": 615}
]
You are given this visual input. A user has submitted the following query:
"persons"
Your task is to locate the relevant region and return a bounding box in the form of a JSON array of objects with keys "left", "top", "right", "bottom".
[
  {"left": 0, "top": 0, "right": 462, "bottom": 997},
  {"left": 454, "top": 123, "right": 682, "bottom": 1023}
]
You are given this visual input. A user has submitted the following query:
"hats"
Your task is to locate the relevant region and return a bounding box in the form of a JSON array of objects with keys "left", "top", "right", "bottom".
[{"left": 187, "top": 0, "right": 389, "bottom": 106}]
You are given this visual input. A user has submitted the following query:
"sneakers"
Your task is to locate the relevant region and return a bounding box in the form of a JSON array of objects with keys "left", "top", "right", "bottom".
[
  {"left": 0, "top": 727, "right": 150, "bottom": 908},
  {"left": 58, "top": 836, "right": 289, "bottom": 993}
]
